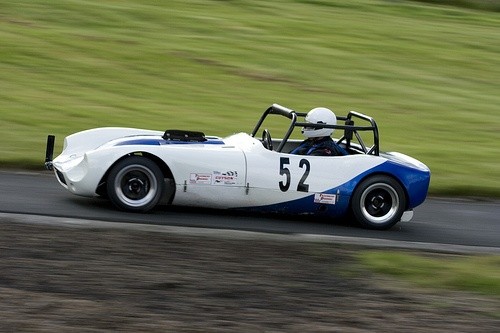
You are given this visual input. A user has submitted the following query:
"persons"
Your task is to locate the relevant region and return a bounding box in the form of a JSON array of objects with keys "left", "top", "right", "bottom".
[{"left": 290, "top": 107, "right": 344, "bottom": 156}]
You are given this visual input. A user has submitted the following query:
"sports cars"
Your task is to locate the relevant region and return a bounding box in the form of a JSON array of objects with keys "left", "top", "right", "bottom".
[{"left": 42, "top": 102, "right": 432, "bottom": 232}]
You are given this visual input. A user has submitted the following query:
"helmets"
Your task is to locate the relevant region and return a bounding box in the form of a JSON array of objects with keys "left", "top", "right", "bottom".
[{"left": 300, "top": 107, "right": 338, "bottom": 138}]
